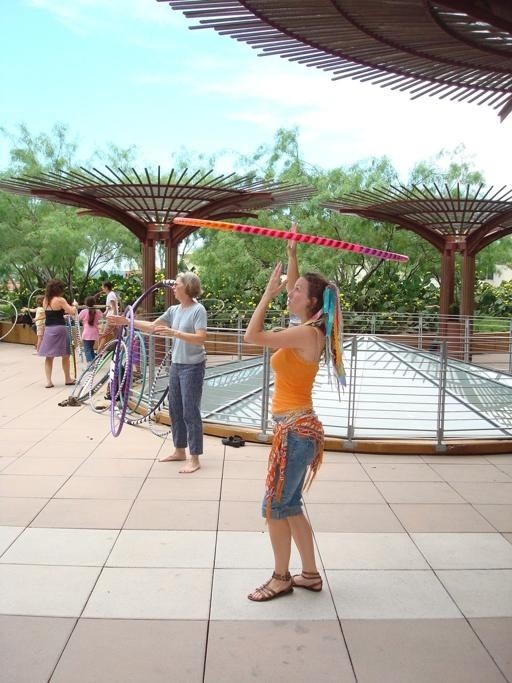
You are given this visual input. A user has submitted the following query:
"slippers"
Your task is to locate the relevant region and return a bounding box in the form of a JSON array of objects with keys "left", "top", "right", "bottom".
[
  {"left": 222, "top": 435, "right": 245, "bottom": 447},
  {"left": 66, "top": 380, "right": 76, "bottom": 385},
  {"left": 45, "top": 385, "right": 54, "bottom": 388}
]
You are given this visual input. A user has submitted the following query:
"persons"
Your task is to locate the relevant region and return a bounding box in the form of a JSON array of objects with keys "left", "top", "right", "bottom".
[
  {"left": 93, "top": 281, "right": 118, "bottom": 356},
  {"left": 107, "top": 272, "right": 207, "bottom": 473},
  {"left": 78, "top": 297, "right": 102, "bottom": 371},
  {"left": 24, "top": 296, "right": 46, "bottom": 353},
  {"left": 38, "top": 279, "right": 78, "bottom": 388},
  {"left": 244, "top": 223, "right": 346, "bottom": 603}
]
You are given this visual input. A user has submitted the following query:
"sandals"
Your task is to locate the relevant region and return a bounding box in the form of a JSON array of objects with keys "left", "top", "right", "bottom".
[{"left": 248, "top": 571, "right": 322, "bottom": 601}]
